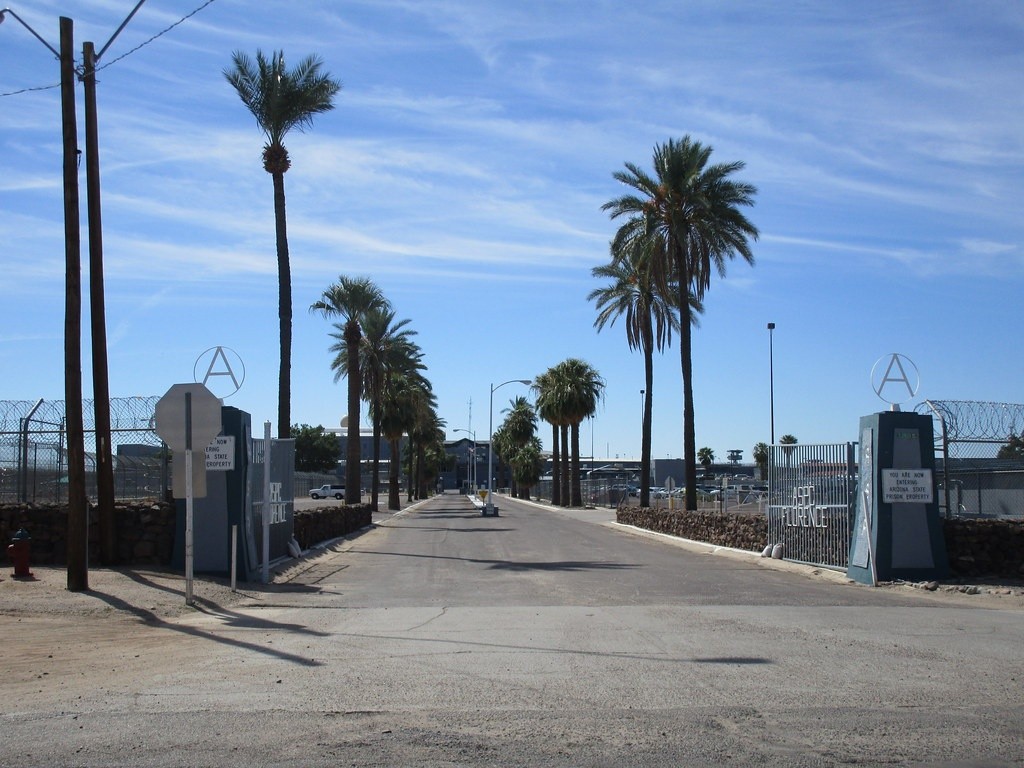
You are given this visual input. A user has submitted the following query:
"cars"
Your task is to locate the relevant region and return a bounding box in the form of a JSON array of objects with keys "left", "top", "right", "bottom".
[{"left": 580, "top": 473, "right": 769, "bottom": 508}]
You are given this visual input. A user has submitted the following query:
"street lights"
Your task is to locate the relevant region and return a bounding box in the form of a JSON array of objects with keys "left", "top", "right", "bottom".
[
  {"left": 482, "top": 378, "right": 534, "bottom": 506},
  {"left": 590, "top": 412, "right": 596, "bottom": 472},
  {"left": 767, "top": 322, "right": 777, "bottom": 443},
  {"left": 639, "top": 388, "right": 648, "bottom": 433},
  {"left": 452, "top": 427, "right": 477, "bottom": 500}
]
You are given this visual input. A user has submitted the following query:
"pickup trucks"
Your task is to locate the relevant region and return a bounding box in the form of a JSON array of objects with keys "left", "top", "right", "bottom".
[{"left": 307, "top": 484, "right": 365, "bottom": 499}]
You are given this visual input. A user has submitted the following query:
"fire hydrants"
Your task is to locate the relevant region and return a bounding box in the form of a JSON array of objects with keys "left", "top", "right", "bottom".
[{"left": 8, "top": 525, "right": 35, "bottom": 578}]
